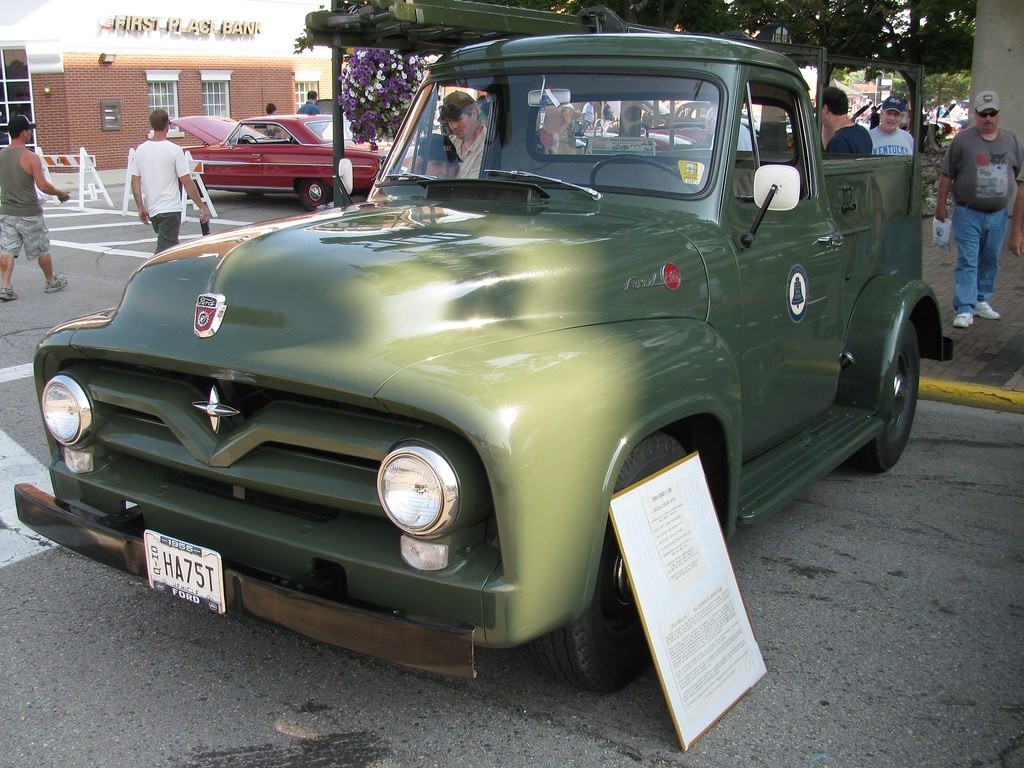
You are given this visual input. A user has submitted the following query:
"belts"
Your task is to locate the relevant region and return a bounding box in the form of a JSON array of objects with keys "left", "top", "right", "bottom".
[{"left": 955, "top": 199, "right": 1002, "bottom": 214}]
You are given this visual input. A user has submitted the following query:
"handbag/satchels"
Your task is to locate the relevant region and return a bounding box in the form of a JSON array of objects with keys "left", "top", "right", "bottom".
[{"left": 933, "top": 216, "right": 952, "bottom": 252}]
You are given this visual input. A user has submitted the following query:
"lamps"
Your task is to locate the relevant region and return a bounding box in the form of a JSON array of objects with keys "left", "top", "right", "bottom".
[
  {"left": 98, "top": 53, "right": 116, "bottom": 62},
  {"left": 43, "top": 82, "right": 51, "bottom": 97}
]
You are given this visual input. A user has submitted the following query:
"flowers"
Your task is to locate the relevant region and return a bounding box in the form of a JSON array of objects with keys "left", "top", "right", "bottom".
[{"left": 338, "top": 48, "right": 431, "bottom": 150}]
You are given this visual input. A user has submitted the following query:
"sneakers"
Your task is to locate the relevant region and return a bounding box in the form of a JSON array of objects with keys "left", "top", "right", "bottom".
[
  {"left": 45, "top": 276, "right": 68, "bottom": 293},
  {"left": 0, "top": 286, "right": 19, "bottom": 300},
  {"left": 973, "top": 302, "right": 1001, "bottom": 319},
  {"left": 952, "top": 312, "right": 973, "bottom": 328}
]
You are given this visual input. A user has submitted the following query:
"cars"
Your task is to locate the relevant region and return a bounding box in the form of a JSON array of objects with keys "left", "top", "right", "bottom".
[
  {"left": 170, "top": 115, "right": 420, "bottom": 212},
  {"left": 850, "top": 94, "right": 961, "bottom": 140},
  {"left": 636, "top": 100, "right": 715, "bottom": 152}
]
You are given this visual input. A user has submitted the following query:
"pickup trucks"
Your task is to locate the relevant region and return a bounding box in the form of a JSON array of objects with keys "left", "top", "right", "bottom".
[{"left": 15, "top": 32, "right": 953, "bottom": 698}]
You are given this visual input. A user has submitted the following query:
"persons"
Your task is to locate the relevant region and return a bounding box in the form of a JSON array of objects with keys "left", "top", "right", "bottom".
[
  {"left": 642, "top": 112, "right": 653, "bottom": 127},
  {"left": 265, "top": 103, "right": 284, "bottom": 139},
  {"left": 603, "top": 101, "right": 613, "bottom": 128},
  {"left": 582, "top": 102, "right": 595, "bottom": 130},
  {"left": 557, "top": 103, "right": 576, "bottom": 155},
  {"left": 821, "top": 87, "right": 873, "bottom": 154},
  {"left": 870, "top": 106, "right": 912, "bottom": 131},
  {"left": 402, "top": 90, "right": 489, "bottom": 179},
  {"left": 934, "top": 92, "right": 1024, "bottom": 327},
  {"left": 932, "top": 100, "right": 963, "bottom": 122},
  {"left": 868, "top": 94, "right": 914, "bottom": 155},
  {"left": 576, "top": 139, "right": 586, "bottom": 155},
  {"left": 1008, "top": 161, "right": 1024, "bottom": 256},
  {"left": 0, "top": 116, "right": 70, "bottom": 300},
  {"left": 297, "top": 91, "right": 321, "bottom": 114},
  {"left": 710, "top": 96, "right": 753, "bottom": 151},
  {"left": 131, "top": 109, "right": 210, "bottom": 256}
]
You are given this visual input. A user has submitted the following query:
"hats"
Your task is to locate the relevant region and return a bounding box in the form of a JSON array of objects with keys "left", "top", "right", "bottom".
[
  {"left": 418, "top": 133, "right": 464, "bottom": 164},
  {"left": 437, "top": 90, "right": 477, "bottom": 122},
  {"left": 975, "top": 91, "right": 1000, "bottom": 112},
  {"left": 8, "top": 115, "right": 36, "bottom": 130},
  {"left": 882, "top": 95, "right": 905, "bottom": 115}
]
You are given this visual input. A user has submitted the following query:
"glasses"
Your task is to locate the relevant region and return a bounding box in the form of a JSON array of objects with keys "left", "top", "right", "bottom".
[
  {"left": 168, "top": 120, "right": 171, "bottom": 125},
  {"left": 976, "top": 110, "right": 999, "bottom": 117}
]
